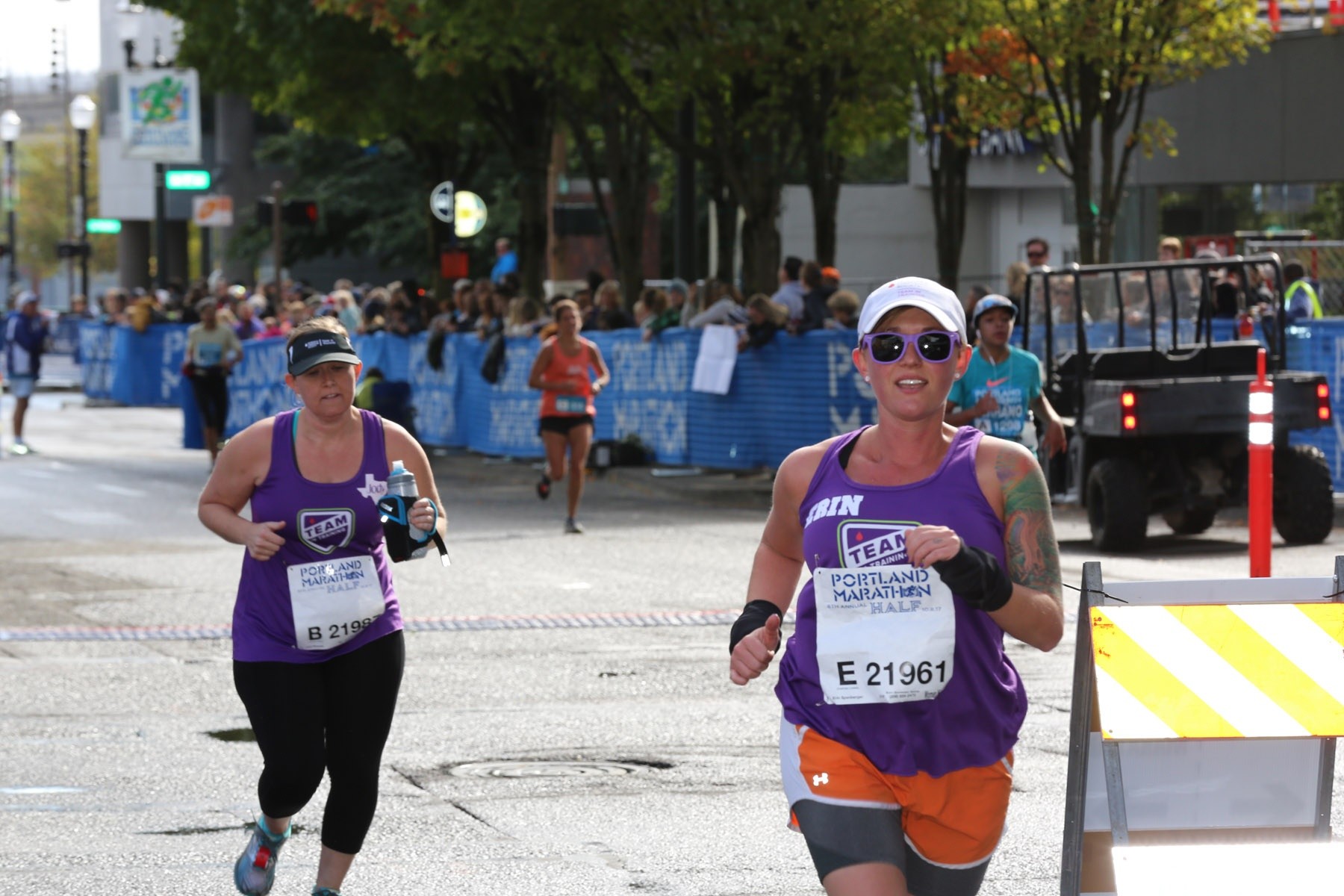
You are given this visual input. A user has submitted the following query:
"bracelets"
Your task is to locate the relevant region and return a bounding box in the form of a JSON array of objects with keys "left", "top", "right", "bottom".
[
  {"left": 727, "top": 595, "right": 786, "bottom": 655},
  {"left": 931, "top": 539, "right": 1015, "bottom": 611}
]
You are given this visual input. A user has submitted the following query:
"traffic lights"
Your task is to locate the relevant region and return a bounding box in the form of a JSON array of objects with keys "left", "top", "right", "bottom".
[{"left": 281, "top": 200, "right": 318, "bottom": 227}]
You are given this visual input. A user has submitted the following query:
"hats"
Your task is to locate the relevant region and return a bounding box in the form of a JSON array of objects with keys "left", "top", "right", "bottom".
[
  {"left": 822, "top": 267, "right": 841, "bottom": 281},
  {"left": 285, "top": 331, "right": 360, "bottom": 376},
  {"left": 15, "top": 291, "right": 37, "bottom": 305},
  {"left": 1161, "top": 237, "right": 1181, "bottom": 251},
  {"left": 858, "top": 277, "right": 968, "bottom": 347}
]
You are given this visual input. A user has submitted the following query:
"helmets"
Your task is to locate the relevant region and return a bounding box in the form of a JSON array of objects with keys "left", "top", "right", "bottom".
[{"left": 972, "top": 294, "right": 1018, "bottom": 326}]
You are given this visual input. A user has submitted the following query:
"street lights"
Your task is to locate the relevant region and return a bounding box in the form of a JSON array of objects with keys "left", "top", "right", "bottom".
[
  {"left": 67, "top": 93, "right": 97, "bottom": 314},
  {"left": 115, "top": 9, "right": 189, "bottom": 324},
  {"left": 0, "top": 107, "right": 23, "bottom": 287}
]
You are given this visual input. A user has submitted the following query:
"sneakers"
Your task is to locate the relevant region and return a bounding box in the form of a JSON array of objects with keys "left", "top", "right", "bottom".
[
  {"left": 312, "top": 890, "right": 341, "bottom": 896},
  {"left": 233, "top": 816, "right": 291, "bottom": 896}
]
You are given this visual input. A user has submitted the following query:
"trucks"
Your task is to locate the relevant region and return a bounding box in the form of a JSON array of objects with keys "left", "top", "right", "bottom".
[{"left": 1019, "top": 249, "right": 1337, "bottom": 553}]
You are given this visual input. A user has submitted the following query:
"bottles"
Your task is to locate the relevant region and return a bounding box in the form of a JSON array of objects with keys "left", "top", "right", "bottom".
[{"left": 387, "top": 460, "right": 427, "bottom": 561}]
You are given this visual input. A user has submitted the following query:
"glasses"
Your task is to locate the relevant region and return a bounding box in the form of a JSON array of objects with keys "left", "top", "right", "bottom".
[
  {"left": 1055, "top": 290, "right": 1069, "bottom": 295},
  {"left": 861, "top": 331, "right": 961, "bottom": 363},
  {"left": 1028, "top": 251, "right": 1043, "bottom": 257},
  {"left": 1163, "top": 247, "right": 1176, "bottom": 252}
]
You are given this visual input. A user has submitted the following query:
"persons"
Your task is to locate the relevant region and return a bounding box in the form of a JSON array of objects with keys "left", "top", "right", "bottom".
[
  {"left": 527, "top": 298, "right": 611, "bottom": 536},
  {"left": 180, "top": 305, "right": 243, "bottom": 465},
  {"left": 944, "top": 293, "right": 1068, "bottom": 464},
  {"left": 728, "top": 277, "right": 1064, "bottom": 896},
  {"left": 97, "top": 236, "right": 1344, "bottom": 334},
  {"left": 197, "top": 316, "right": 448, "bottom": 896},
  {"left": 356, "top": 367, "right": 382, "bottom": 413},
  {"left": 0, "top": 291, "right": 51, "bottom": 456},
  {"left": 58, "top": 296, "right": 93, "bottom": 361}
]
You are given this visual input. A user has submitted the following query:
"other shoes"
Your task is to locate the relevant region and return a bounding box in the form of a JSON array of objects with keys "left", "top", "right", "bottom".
[
  {"left": 567, "top": 521, "right": 582, "bottom": 531},
  {"left": 10, "top": 442, "right": 29, "bottom": 453},
  {"left": 538, "top": 478, "right": 550, "bottom": 498}
]
